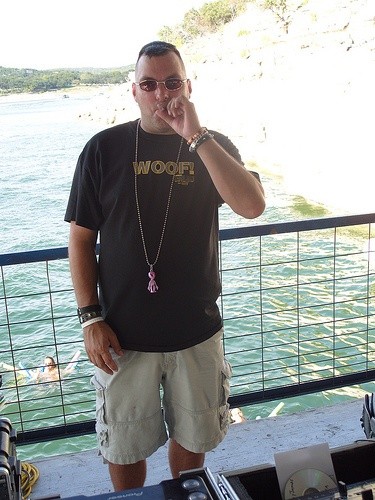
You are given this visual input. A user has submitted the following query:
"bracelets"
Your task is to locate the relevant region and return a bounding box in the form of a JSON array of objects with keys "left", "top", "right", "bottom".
[
  {"left": 186, "top": 127, "right": 213, "bottom": 153},
  {"left": 76, "top": 305, "right": 104, "bottom": 328}
]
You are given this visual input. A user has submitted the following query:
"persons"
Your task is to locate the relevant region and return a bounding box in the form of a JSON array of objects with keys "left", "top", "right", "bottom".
[
  {"left": 64, "top": 41, "right": 265, "bottom": 491},
  {"left": 35, "top": 356, "right": 61, "bottom": 380}
]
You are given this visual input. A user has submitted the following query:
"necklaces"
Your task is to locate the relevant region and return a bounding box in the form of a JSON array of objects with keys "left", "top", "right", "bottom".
[{"left": 136, "top": 119, "right": 183, "bottom": 292}]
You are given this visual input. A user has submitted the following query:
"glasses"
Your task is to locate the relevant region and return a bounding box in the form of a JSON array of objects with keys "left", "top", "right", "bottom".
[{"left": 134, "top": 78, "right": 188, "bottom": 92}]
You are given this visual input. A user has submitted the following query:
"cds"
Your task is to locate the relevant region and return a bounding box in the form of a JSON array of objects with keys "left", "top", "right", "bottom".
[{"left": 284, "top": 469, "right": 340, "bottom": 500}]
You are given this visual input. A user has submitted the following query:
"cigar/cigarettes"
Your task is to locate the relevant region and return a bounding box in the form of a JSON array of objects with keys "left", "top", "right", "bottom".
[{"left": 162, "top": 105, "right": 166, "bottom": 112}]
